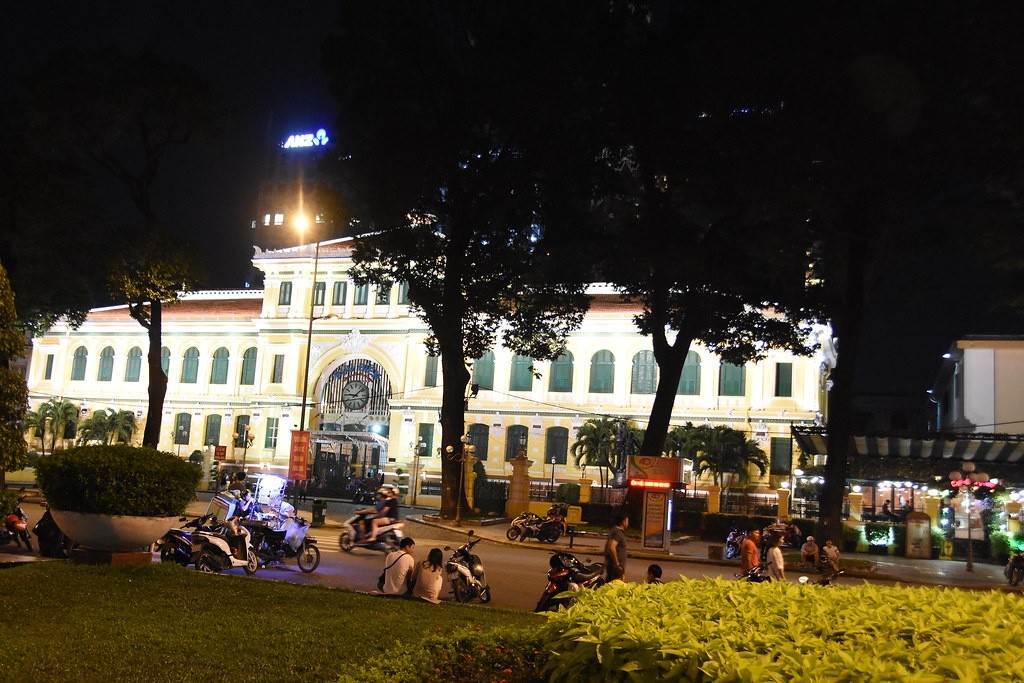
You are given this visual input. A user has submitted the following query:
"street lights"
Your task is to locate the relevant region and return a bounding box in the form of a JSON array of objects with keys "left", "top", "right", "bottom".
[
  {"left": 446, "top": 434, "right": 477, "bottom": 527},
  {"left": 550, "top": 453, "right": 557, "bottom": 492},
  {"left": 170, "top": 425, "right": 187, "bottom": 456},
  {"left": 410, "top": 435, "right": 427, "bottom": 506},
  {"left": 291, "top": 212, "right": 325, "bottom": 431},
  {"left": 581, "top": 462, "right": 586, "bottom": 479},
  {"left": 949, "top": 462, "right": 990, "bottom": 571},
  {"left": 232, "top": 425, "right": 256, "bottom": 472}
]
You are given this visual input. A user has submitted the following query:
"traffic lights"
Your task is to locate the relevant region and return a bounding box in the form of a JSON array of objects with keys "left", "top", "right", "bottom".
[{"left": 210, "top": 469, "right": 218, "bottom": 480}]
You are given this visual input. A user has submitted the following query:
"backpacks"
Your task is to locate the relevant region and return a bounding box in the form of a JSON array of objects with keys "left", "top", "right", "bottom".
[{"left": 219, "top": 474, "right": 227, "bottom": 485}]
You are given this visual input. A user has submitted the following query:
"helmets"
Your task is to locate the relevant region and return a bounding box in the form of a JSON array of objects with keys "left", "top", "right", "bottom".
[
  {"left": 387, "top": 486, "right": 399, "bottom": 496},
  {"left": 376, "top": 488, "right": 388, "bottom": 496}
]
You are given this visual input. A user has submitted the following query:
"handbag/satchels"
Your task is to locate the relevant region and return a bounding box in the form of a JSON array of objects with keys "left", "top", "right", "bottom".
[
  {"left": 283, "top": 516, "right": 311, "bottom": 552},
  {"left": 378, "top": 570, "right": 386, "bottom": 582}
]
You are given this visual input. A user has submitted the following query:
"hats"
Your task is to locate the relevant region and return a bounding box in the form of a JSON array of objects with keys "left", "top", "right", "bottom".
[{"left": 806, "top": 536, "right": 813, "bottom": 541}]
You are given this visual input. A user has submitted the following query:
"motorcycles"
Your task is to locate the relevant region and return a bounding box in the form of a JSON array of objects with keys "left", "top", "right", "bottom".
[
  {"left": 443, "top": 530, "right": 490, "bottom": 605},
  {"left": 506, "top": 504, "right": 569, "bottom": 543},
  {"left": 191, "top": 516, "right": 257, "bottom": 574},
  {"left": 352, "top": 486, "right": 378, "bottom": 504},
  {"left": 0, "top": 495, "right": 34, "bottom": 553},
  {"left": 734, "top": 561, "right": 773, "bottom": 584},
  {"left": 534, "top": 549, "right": 606, "bottom": 614},
  {"left": 154, "top": 512, "right": 217, "bottom": 567},
  {"left": 724, "top": 526, "right": 745, "bottom": 559},
  {"left": 241, "top": 515, "right": 320, "bottom": 573},
  {"left": 1004, "top": 547, "right": 1024, "bottom": 586},
  {"left": 763, "top": 522, "right": 801, "bottom": 549},
  {"left": 339, "top": 509, "right": 405, "bottom": 553},
  {"left": 799, "top": 567, "right": 845, "bottom": 588}
]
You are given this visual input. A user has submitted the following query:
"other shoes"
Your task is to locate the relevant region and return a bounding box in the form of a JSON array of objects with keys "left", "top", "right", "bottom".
[
  {"left": 366, "top": 538, "right": 376, "bottom": 543},
  {"left": 814, "top": 566, "right": 819, "bottom": 571},
  {"left": 354, "top": 536, "right": 365, "bottom": 541}
]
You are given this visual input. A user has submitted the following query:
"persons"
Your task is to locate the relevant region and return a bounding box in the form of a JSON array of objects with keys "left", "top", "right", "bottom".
[
  {"left": 354, "top": 479, "right": 398, "bottom": 542},
  {"left": 882, "top": 499, "right": 912, "bottom": 524},
  {"left": 220, "top": 471, "right": 308, "bottom": 522},
  {"left": 646, "top": 564, "right": 666, "bottom": 584},
  {"left": 800, "top": 536, "right": 819, "bottom": 572},
  {"left": 604, "top": 511, "right": 629, "bottom": 583},
  {"left": 383, "top": 537, "right": 415, "bottom": 595},
  {"left": 823, "top": 538, "right": 845, "bottom": 574},
  {"left": 767, "top": 531, "right": 785, "bottom": 582},
  {"left": 409, "top": 548, "right": 443, "bottom": 600},
  {"left": 741, "top": 524, "right": 760, "bottom": 577}
]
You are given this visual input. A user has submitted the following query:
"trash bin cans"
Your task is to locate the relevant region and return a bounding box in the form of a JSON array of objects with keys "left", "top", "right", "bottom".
[{"left": 312, "top": 499, "right": 327, "bottom": 522}]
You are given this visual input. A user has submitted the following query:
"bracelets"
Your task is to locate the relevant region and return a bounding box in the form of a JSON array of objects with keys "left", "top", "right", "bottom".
[{"left": 615, "top": 564, "right": 620, "bottom": 566}]
